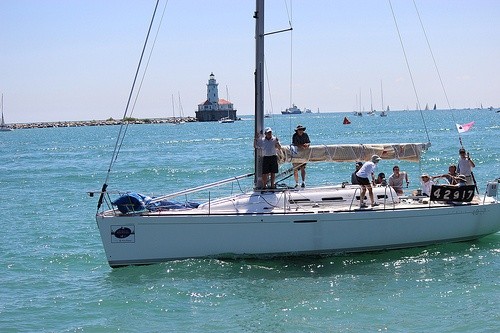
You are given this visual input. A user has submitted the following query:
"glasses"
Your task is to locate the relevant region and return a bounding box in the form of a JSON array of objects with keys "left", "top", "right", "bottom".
[
  {"left": 460, "top": 151, "right": 465, "bottom": 154},
  {"left": 422, "top": 177, "right": 425, "bottom": 178}
]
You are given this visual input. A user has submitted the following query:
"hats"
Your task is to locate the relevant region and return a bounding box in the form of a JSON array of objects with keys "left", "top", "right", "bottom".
[
  {"left": 459, "top": 148, "right": 465, "bottom": 153},
  {"left": 378, "top": 173, "right": 385, "bottom": 178},
  {"left": 294, "top": 125, "right": 306, "bottom": 133},
  {"left": 356, "top": 162, "right": 363, "bottom": 166},
  {"left": 265, "top": 127, "right": 272, "bottom": 133},
  {"left": 372, "top": 155, "right": 382, "bottom": 160},
  {"left": 420, "top": 173, "right": 430, "bottom": 179}
]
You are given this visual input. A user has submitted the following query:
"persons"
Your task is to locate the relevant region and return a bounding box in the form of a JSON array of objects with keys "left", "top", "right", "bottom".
[
  {"left": 431, "top": 164, "right": 459, "bottom": 185},
  {"left": 372, "top": 173, "right": 388, "bottom": 187},
  {"left": 352, "top": 162, "right": 363, "bottom": 185},
  {"left": 356, "top": 154, "right": 382, "bottom": 208},
  {"left": 455, "top": 175, "right": 466, "bottom": 186},
  {"left": 412, "top": 174, "right": 431, "bottom": 201},
  {"left": 262, "top": 127, "right": 281, "bottom": 189},
  {"left": 292, "top": 125, "right": 312, "bottom": 188},
  {"left": 457, "top": 148, "right": 476, "bottom": 185},
  {"left": 389, "top": 166, "right": 410, "bottom": 196}
]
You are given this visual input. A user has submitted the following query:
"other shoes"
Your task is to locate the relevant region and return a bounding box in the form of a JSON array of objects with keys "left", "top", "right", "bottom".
[
  {"left": 371, "top": 202, "right": 380, "bottom": 207},
  {"left": 360, "top": 203, "right": 368, "bottom": 208},
  {"left": 301, "top": 183, "right": 305, "bottom": 187},
  {"left": 295, "top": 184, "right": 298, "bottom": 188}
]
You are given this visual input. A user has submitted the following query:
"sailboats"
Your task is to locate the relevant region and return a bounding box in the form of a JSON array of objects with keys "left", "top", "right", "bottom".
[
  {"left": 0, "top": 92, "right": 12, "bottom": 131},
  {"left": 170, "top": 90, "right": 186, "bottom": 125},
  {"left": 218, "top": 83, "right": 234, "bottom": 123},
  {"left": 90, "top": 0, "right": 500, "bottom": 269},
  {"left": 352, "top": 86, "right": 377, "bottom": 117},
  {"left": 379, "top": 79, "right": 388, "bottom": 117}
]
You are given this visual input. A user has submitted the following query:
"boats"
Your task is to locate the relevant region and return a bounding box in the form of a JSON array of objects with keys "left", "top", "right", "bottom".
[{"left": 280, "top": 103, "right": 302, "bottom": 115}]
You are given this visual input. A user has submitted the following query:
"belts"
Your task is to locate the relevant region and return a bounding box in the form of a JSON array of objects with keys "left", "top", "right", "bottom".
[{"left": 466, "top": 176, "right": 471, "bottom": 177}]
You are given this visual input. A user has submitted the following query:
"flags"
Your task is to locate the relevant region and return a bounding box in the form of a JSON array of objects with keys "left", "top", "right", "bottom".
[{"left": 456, "top": 120, "right": 475, "bottom": 133}]
